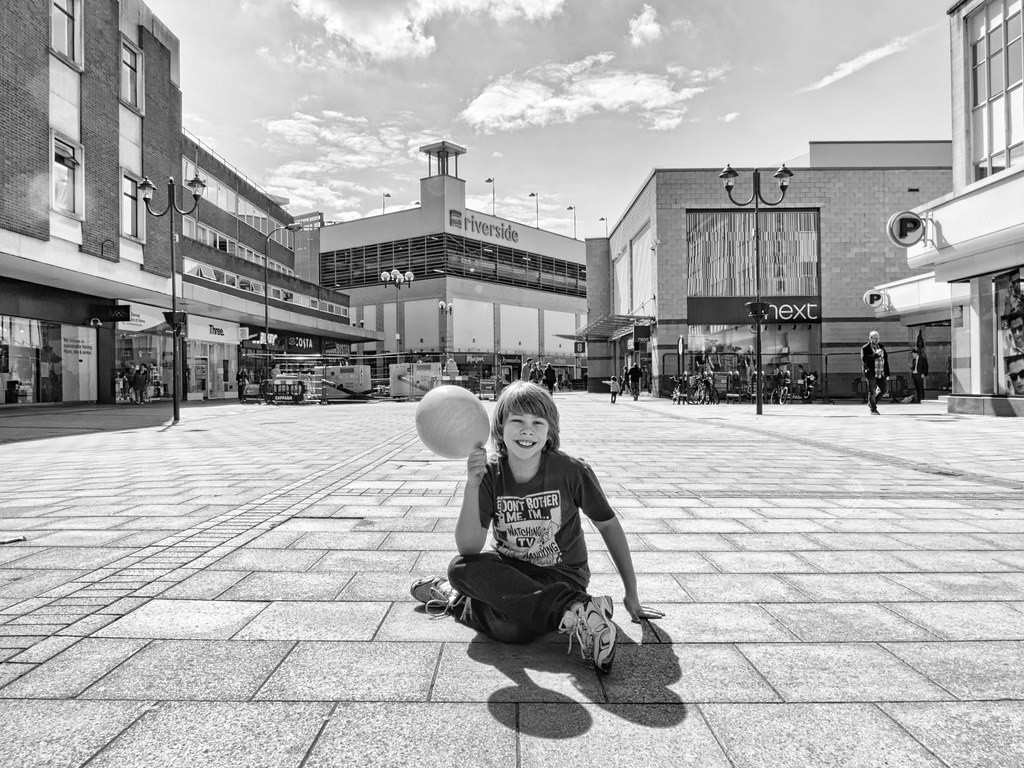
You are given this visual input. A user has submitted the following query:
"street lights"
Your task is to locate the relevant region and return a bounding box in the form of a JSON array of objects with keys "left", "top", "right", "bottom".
[
  {"left": 439, "top": 301, "right": 455, "bottom": 361},
  {"left": 721, "top": 164, "right": 796, "bottom": 414},
  {"left": 486, "top": 177, "right": 494, "bottom": 216},
  {"left": 136, "top": 175, "right": 206, "bottom": 421},
  {"left": 381, "top": 270, "right": 415, "bottom": 363},
  {"left": 599, "top": 217, "right": 607, "bottom": 236},
  {"left": 382, "top": 194, "right": 391, "bottom": 214},
  {"left": 566, "top": 206, "right": 576, "bottom": 238},
  {"left": 529, "top": 192, "right": 539, "bottom": 229},
  {"left": 264, "top": 223, "right": 302, "bottom": 404}
]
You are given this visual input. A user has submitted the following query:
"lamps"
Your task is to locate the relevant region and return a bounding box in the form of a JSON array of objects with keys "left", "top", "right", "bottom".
[{"left": 90, "top": 318, "right": 102, "bottom": 326}]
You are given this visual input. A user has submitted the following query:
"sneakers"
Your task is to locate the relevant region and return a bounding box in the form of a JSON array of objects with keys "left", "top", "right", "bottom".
[
  {"left": 559, "top": 596, "right": 616, "bottom": 679},
  {"left": 409, "top": 575, "right": 466, "bottom": 617}
]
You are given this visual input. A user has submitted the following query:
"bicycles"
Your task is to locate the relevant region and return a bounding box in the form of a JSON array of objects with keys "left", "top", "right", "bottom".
[
  {"left": 770, "top": 367, "right": 788, "bottom": 404},
  {"left": 670, "top": 376, "right": 720, "bottom": 404}
]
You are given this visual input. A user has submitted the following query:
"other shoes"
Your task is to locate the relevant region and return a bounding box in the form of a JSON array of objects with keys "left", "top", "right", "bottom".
[
  {"left": 871, "top": 411, "right": 880, "bottom": 415},
  {"left": 135, "top": 402, "right": 144, "bottom": 404}
]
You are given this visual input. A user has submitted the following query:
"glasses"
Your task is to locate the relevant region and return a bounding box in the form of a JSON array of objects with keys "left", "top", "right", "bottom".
[
  {"left": 1012, "top": 277, "right": 1020, "bottom": 285},
  {"left": 1009, "top": 369, "right": 1024, "bottom": 381},
  {"left": 1011, "top": 324, "right": 1024, "bottom": 334}
]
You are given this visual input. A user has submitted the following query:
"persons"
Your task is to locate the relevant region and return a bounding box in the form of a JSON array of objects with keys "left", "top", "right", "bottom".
[
  {"left": 695, "top": 345, "right": 755, "bottom": 383},
  {"left": 132, "top": 363, "right": 149, "bottom": 405},
  {"left": 123, "top": 375, "right": 129, "bottom": 399},
  {"left": 521, "top": 359, "right": 648, "bottom": 404},
  {"left": 860, "top": 331, "right": 890, "bottom": 415},
  {"left": 1004, "top": 269, "right": 1024, "bottom": 395},
  {"left": 236, "top": 365, "right": 251, "bottom": 403},
  {"left": 907, "top": 349, "right": 928, "bottom": 404},
  {"left": 410, "top": 381, "right": 666, "bottom": 673}
]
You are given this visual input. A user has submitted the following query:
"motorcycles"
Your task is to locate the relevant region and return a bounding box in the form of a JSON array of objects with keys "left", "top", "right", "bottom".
[{"left": 797, "top": 365, "right": 818, "bottom": 404}]
